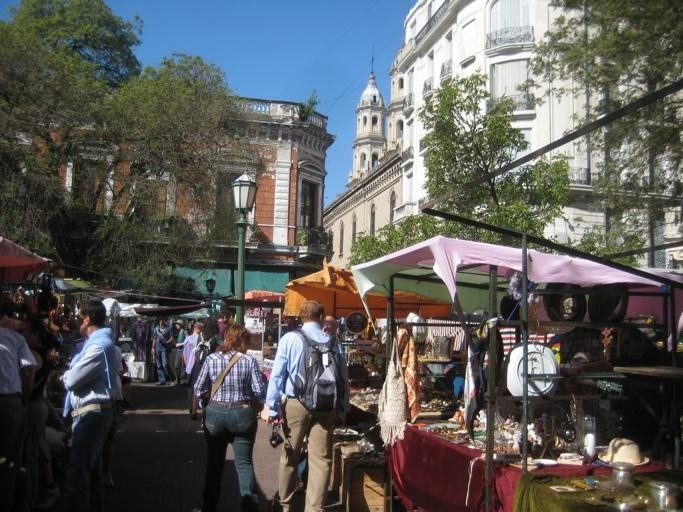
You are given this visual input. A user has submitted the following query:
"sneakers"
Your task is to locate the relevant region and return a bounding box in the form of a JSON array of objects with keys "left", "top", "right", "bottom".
[{"left": 38, "top": 486, "right": 62, "bottom": 509}]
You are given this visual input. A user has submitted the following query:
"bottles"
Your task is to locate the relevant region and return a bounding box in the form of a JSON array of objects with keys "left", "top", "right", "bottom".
[
  {"left": 611, "top": 461, "right": 635, "bottom": 486},
  {"left": 648, "top": 480, "right": 679, "bottom": 512}
]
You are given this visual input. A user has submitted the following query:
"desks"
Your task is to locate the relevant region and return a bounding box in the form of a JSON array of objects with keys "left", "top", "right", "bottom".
[{"left": 350, "top": 385, "right": 683, "bottom": 512}]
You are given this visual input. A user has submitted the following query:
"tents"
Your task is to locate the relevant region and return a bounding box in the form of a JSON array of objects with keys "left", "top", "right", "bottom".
[{"left": 244, "top": 289, "right": 284, "bottom": 299}]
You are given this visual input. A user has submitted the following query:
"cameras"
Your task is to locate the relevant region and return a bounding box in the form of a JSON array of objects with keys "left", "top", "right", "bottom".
[{"left": 269, "top": 434, "right": 283, "bottom": 449}]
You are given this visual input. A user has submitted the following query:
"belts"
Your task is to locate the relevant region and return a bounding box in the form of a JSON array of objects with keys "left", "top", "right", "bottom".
[
  {"left": 70, "top": 401, "right": 112, "bottom": 418},
  {"left": 208, "top": 399, "right": 252, "bottom": 407}
]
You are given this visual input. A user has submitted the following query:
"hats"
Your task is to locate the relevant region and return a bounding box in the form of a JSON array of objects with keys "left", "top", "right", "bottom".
[{"left": 174, "top": 319, "right": 187, "bottom": 329}]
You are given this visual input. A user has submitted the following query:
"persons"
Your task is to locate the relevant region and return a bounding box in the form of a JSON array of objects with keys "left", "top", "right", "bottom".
[
  {"left": 128, "top": 316, "right": 203, "bottom": 387},
  {"left": 1, "top": 287, "right": 116, "bottom": 510},
  {"left": 184, "top": 301, "right": 351, "bottom": 510}
]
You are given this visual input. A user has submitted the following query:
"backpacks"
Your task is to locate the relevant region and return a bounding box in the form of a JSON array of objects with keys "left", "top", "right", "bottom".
[
  {"left": 188, "top": 335, "right": 215, "bottom": 390},
  {"left": 288, "top": 328, "right": 345, "bottom": 412}
]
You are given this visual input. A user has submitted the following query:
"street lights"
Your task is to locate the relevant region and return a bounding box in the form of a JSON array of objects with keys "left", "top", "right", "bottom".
[{"left": 231, "top": 168, "right": 259, "bottom": 325}]
